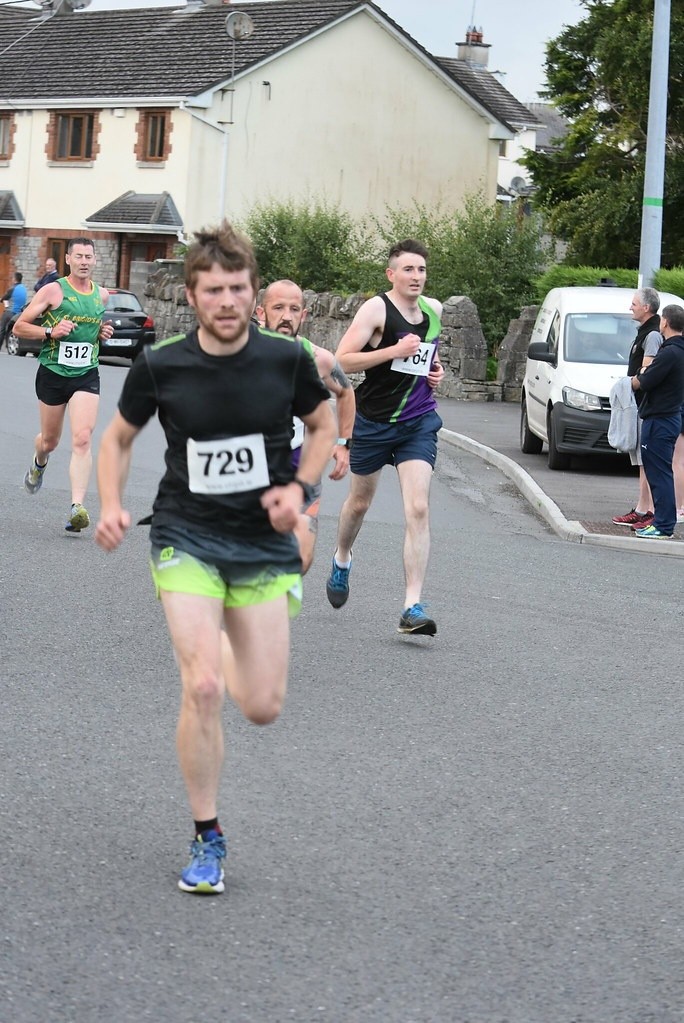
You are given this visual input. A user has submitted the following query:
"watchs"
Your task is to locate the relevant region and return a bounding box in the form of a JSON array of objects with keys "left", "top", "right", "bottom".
[
  {"left": 45, "top": 327, "right": 52, "bottom": 339},
  {"left": 434, "top": 361, "right": 445, "bottom": 371},
  {"left": 293, "top": 477, "right": 317, "bottom": 508},
  {"left": 337, "top": 437, "right": 353, "bottom": 449}
]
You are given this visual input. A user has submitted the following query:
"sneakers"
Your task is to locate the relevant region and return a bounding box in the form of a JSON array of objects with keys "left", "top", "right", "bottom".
[
  {"left": 23, "top": 453, "right": 52, "bottom": 494},
  {"left": 177, "top": 833, "right": 228, "bottom": 893},
  {"left": 631, "top": 511, "right": 656, "bottom": 531},
  {"left": 635, "top": 522, "right": 674, "bottom": 540},
  {"left": 612, "top": 509, "right": 644, "bottom": 526},
  {"left": 64, "top": 506, "right": 90, "bottom": 533},
  {"left": 326, "top": 545, "right": 352, "bottom": 609},
  {"left": 399, "top": 609, "right": 437, "bottom": 637}
]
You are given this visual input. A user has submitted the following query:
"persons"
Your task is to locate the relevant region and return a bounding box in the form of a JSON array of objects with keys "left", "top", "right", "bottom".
[
  {"left": 0, "top": 272, "right": 27, "bottom": 353},
  {"left": 613, "top": 287, "right": 684, "bottom": 540},
  {"left": 34, "top": 258, "right": 59, "bottom": 294},
  {"left": 256, "top": 279, "right": 355, "bottom": 580},
  {"left": 12, "top": 238, "right": 114, "bottom": 533},
  {"left": 326, "top": 239, "right": 443, "bottom": 636},
  {"left": 93, "top": 226, "right": 337, "bottom": 893}
]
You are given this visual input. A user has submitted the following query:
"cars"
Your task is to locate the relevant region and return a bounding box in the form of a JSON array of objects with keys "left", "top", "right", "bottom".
[{"left": 5, "top": 287, "right": 156, "bottom": 366}]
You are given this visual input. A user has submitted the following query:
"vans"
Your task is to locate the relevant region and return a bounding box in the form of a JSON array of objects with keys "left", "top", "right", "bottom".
[{"left": 518, "top": 286, "right": 684, "bottom": 469}]
